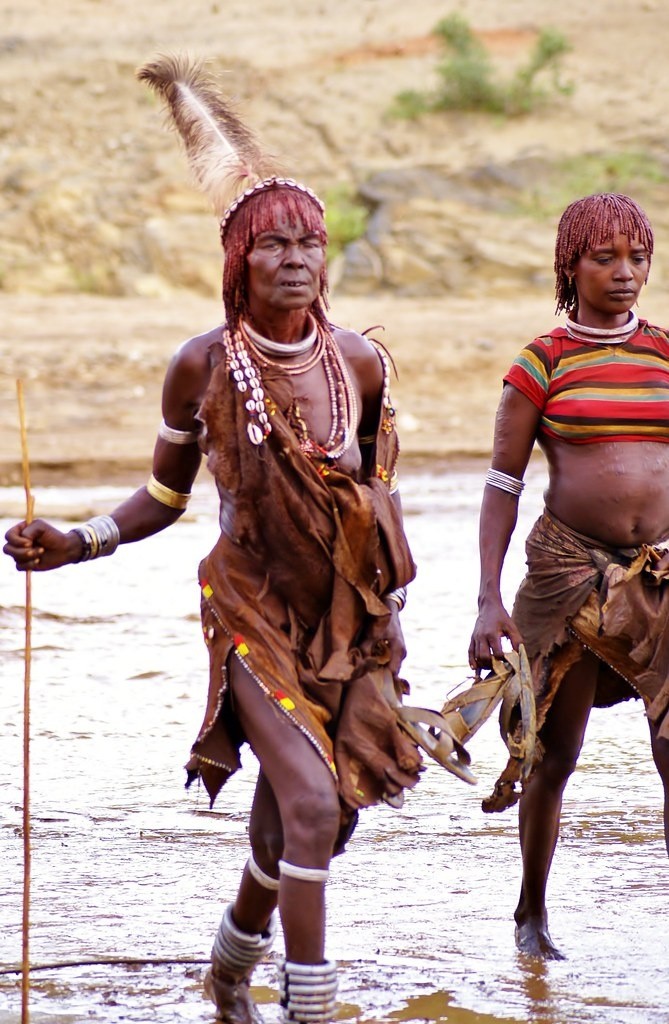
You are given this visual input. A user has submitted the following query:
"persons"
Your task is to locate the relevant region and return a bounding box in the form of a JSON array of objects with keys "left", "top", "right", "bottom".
[
  {"left": 467, "top": 193, "right": 669, "bottom": 960},
  {"left": 2, "top": 177, "right": 409, "bottom": 1024}
]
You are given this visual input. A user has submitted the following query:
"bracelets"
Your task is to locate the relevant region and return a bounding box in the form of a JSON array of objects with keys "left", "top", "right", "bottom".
[
  {"left": 70, "top": 515, "right": 120, "bottom": 563},
  {"left": 386, "top": 586, "right": 407, "bottom": 609}
]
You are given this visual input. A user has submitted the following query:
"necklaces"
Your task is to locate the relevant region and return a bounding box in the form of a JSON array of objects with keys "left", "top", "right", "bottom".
[
  {"left": 237, "top": 311, "right": 358, "bottom": 458},
  {"left": 564, "top": 309, "right": 638, "bottom": 343}
]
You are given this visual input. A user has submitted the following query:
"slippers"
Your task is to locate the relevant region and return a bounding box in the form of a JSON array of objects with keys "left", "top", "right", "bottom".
[
  {"left": 497, "top": 643, "right": 538, "bottom": 779},
  {"left": 428, "top": 652, "right": 514, "bottom": 753},
  {"left": 366, "top": 667, "right": 476, "bottom": 810}
]
